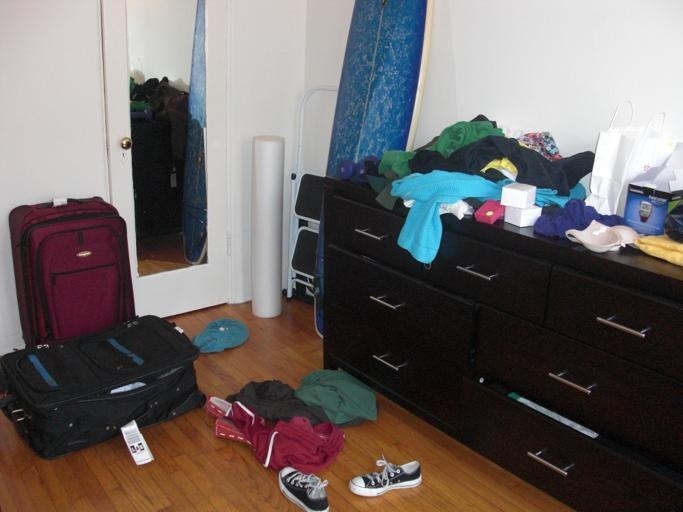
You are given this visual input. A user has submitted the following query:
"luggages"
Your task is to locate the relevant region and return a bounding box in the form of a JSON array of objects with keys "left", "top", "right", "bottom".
[{"left": 0, "top": 197, "right": 207, "bottom": 460}]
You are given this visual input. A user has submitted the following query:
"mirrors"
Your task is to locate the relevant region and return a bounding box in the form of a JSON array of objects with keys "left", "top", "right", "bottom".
[{"left": 125, "top": 1, "right": 209, "bottom": 277}]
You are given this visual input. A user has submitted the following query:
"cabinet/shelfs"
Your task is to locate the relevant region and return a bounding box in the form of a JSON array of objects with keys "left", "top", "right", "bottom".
[
  {"left": 323, "top": 177, "right": 683, "bottom": 512},
  {"left": 129, "top": 111, "right": 188, "bottom": 239}
]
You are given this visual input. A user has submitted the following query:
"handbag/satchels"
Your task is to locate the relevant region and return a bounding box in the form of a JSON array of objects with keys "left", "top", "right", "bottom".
[{"left": 591, "top": 101, "right": 677, "bottom": 217}]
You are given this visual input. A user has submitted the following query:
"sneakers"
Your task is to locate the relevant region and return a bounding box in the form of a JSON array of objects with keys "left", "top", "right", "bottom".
[
  {"left": 349, "top": 460, "right": 423, "bottom": 498},
  {"left": 278, "top": 467, "right": 329, "bottom": 512}
]
